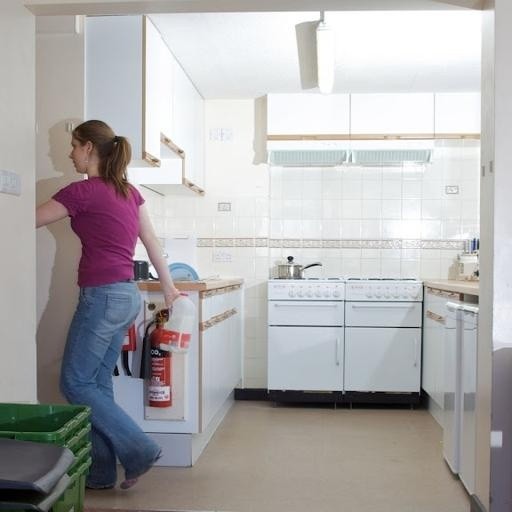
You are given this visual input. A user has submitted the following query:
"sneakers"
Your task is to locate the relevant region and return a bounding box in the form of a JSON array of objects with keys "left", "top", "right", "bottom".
[{"left": 121, "top": 443, "right": 164, "bottom": 493}]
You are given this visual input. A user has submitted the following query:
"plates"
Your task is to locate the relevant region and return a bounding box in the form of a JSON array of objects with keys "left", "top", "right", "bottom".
[{"left": 169, "top": 263, "right": 198, "bottom": 281}]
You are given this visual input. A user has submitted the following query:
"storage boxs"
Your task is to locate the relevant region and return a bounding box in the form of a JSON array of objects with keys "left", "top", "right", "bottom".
[{"left": 0, "top": 399, "right": 97, "bottom": 512}]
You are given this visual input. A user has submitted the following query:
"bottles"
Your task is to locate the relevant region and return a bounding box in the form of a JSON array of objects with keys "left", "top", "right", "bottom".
[
  {"left": 135, "top": 261, "right": 149, "bottom": 280},
  {"left": 457, "top": 237, "right": 480, "bottom": 279}
]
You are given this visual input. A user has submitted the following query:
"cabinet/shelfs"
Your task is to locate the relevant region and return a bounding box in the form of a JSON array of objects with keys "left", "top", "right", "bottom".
[
  {"left": 262, "top": 91, "right": 350, "bottom": 154},
  {"left": 100, "top": 276, "right": 244, "bottom": 472},
  {"left": 421, "top": 289, "right": 478, "bottom": 429},
  {"left": 344, "top": 92, "right": 436, "bottom": 151},
  {"left": 432, "top": 90, "right": 482, "bottom": 142},
  {"left": 81, "top": 15, "right": 205, "bottom": 201}
]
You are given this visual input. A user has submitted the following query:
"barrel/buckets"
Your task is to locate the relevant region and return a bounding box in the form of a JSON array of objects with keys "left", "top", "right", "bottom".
[{"left": 159, "top": 292, "right": 195, "bottom": 355}]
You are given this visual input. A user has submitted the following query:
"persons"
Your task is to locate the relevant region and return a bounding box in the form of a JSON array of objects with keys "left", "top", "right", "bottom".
[{"left": 36, "top": 120, "right": 179, "bottom": 492}]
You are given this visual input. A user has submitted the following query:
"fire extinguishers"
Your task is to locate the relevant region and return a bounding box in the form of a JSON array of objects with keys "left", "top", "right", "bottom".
[
  {"left": 139, "top": 308, "right": 172, "bottom": 407},
  {"left": 121, "top": 323, "right": 136, "bottom": 351}
]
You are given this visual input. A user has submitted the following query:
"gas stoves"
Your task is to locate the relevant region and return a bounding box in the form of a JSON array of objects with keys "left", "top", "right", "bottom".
[{"left": 269, "top": 277, "right": 422, "bottom": 300}]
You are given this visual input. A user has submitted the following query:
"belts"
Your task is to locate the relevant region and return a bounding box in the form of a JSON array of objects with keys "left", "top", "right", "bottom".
[{"left": 119, "top": 279, "right": 136, "bottom": 283}]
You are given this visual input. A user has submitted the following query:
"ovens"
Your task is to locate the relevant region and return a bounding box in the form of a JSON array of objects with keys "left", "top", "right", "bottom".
[{"left": 442, "top": 302, "right": 478, "bottom": 495}]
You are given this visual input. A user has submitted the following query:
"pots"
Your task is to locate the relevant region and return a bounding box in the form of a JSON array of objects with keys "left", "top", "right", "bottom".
[{"left": 276, "top": 256, "right": 321, "bottom": 279}]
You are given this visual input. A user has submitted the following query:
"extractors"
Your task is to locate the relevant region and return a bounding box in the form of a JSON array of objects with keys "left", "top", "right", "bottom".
[{"left": 266, "top": 148, "right": 433, "bottom": 167}]
